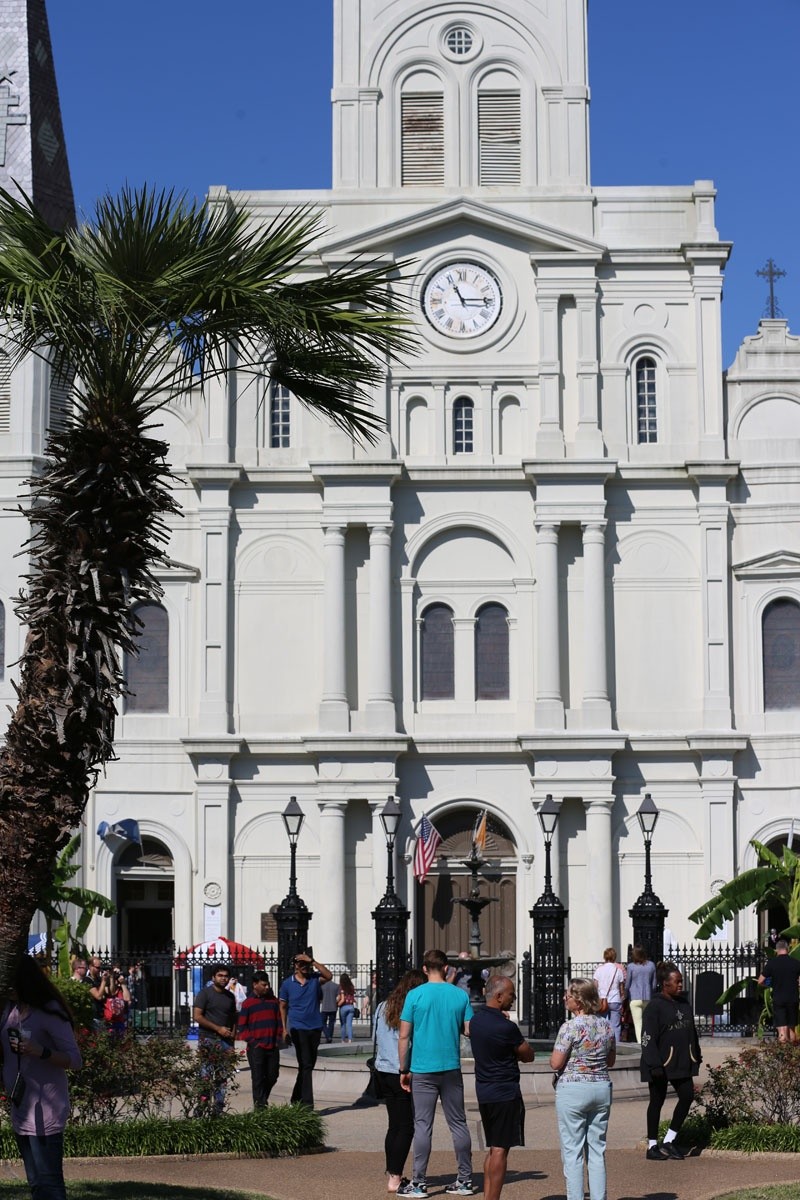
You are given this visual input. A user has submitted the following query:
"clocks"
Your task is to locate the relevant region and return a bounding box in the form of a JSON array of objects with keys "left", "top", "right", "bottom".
[{"left": 421, "top": 260, "right": 502, "bottom": 339}]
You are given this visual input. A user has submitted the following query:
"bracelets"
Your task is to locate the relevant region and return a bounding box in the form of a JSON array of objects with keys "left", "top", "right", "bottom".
[
  {"left": 309, "top": 959, "right": 314, "bottom": 963},
  {"left": 399, "top": 1069, "right": 409, "bottom": 1074}
]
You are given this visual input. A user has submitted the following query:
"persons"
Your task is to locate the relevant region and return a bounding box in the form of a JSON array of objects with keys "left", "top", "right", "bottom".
[
  {"left": 0, "top": 953, "right": 82, "bottom": 1200},
  {"left": 371, "top": 968, "right": 429, "bottom": 1192},
  {"left": 593, "top": 948, "right": 627, "bottom": 1043},
  {"left": 768, "top": 928, "right": 779, "bottom": 950},
  {"left": 37, "top": 952, "right": 143, "bottom": 1065},
  {"left": 759, "top": 940, "right": 800, "bottom": 1041},
  {"left": 549, "top": 977, "right": 617, "bottom": 1200},
  {"left": 624, "top": 944, "right": 656, "bottom": 1044},
  {"left": 189, "top": 950, "right": 377, "bottom": 1118},
  {"left": 639, "top": 962, "right": 700, "bottom": 1161},
  {"left": 395, "top": 949, "right": 535, "bottom": 1200}
]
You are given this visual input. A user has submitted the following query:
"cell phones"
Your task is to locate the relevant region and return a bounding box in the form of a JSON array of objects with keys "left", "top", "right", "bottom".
[{"left": 7, "top": 1028, "right": 23, "bottom": 1055}]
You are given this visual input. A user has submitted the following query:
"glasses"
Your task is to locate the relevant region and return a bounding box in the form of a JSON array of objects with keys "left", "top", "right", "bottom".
[{"left": 563, "top": 991, "right": 573, "bottom": 1002}]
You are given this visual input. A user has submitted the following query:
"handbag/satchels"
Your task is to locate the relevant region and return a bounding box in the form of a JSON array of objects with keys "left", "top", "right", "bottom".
[
  {"left": 364, "top": 1001, "right": 384, "bottom": 1101},
  {"left": 598, "top": 998, "right": 608, "bottom": 1013}
]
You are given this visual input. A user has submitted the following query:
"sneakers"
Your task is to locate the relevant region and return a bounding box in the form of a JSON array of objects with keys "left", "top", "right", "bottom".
[
  {"left": 444, "top": 1179, "right": 474, "bottom": 1195},
  {"left": 396, "top": 1180, "right": 428, "bottom": 1198}
]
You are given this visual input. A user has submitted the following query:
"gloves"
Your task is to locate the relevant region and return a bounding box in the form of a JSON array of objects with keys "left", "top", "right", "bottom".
[{"left": 650, "top": 1066, "right": 667, "bottom": 1088}]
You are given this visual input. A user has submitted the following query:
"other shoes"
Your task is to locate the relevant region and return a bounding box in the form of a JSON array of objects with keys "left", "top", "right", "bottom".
[
  {"left": 646, "top": 1146, "right": 668, "bottom": 1161},
  {"left": 659, "top": 1139, "right": 684, "bottom": 1159},
  {"left": 387, "top": 1177, "right": 411, "bottom": 1194}
]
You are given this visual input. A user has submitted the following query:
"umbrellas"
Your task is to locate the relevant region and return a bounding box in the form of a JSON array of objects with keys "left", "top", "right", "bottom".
[{"left": 172, "top": 936, "right": 265, "bottom": 970}]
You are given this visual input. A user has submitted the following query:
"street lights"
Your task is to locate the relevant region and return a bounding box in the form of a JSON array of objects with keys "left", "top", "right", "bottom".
[
  {"left": 272, "top": 796, "right": 313, "bottom": 1002},
  {"left": 370, "top": 796, "right": 412, "bottom": 1010},
  {"left": 628, "top": 792, "right": 669, "bottom": 967},
  {"left": 527, "top": 793, "right": 570, "bottom": 1039}
]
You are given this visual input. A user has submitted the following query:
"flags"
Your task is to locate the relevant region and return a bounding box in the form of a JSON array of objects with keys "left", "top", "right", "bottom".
[
  {"left": 466, "top": 813, "right": 487, "bottom": 859},
  {"left": 412, "top": 815, "right": 440, "bottom": 885},
  {"left": 97, "top": 815, "right": 142, "bottom": 843}
]
